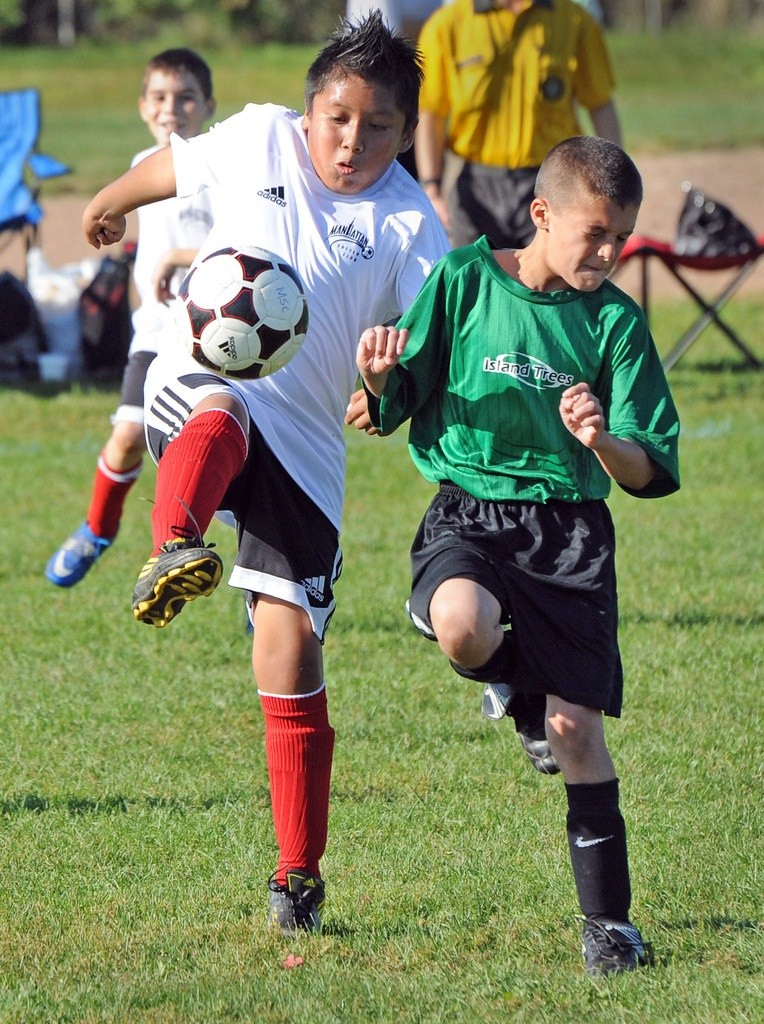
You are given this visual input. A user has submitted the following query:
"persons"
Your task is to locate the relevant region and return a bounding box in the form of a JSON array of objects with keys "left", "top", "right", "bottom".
[
  {"left": 411, "top": 1, "right": 625, "bottom": 256},
  {"left": 47, "top": 48, "right": 216, "bottom": 590},
  {"left": 84, "top": 10, "right": 454, "bottom": 933},
  {"left": 352, "top": 135, "right": 686, "bottom": 972}
]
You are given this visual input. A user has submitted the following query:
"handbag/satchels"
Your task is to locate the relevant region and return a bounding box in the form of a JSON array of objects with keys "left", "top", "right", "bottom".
[{"left": 672, "top": 189, "right": 756, "bottom": 255}]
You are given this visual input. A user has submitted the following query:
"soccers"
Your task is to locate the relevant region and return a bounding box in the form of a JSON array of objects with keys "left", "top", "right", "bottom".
[{"left": 174, "top": 244, "right": 310, "bottom": 380}]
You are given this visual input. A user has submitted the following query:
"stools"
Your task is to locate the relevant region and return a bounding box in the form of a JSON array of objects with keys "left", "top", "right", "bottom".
[{"left": 628, "top": 233, "right": 764, "bottom": 374}]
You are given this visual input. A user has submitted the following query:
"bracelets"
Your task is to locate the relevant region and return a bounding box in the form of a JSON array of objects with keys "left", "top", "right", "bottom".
[{"left": 417, "top": 177, "right": 442, "bottom": 186}]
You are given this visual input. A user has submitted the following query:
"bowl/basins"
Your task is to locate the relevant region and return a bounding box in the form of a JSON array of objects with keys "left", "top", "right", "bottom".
[{"left": 38, "top": 354, "right": 70, "bottom": 383}]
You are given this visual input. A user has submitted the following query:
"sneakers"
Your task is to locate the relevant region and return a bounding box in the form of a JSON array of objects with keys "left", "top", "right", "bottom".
[
  {"left": 45, "top": 522, "right": 110, "bottom": 588},
  {"left": 579, "top": 915, "right": 655, "bottom": 980},
  {"left": 131, "top": 538, "right": 222, "bottom": 629},
  {"left": 266, "top": 870, "right": 326, "bottom": 936},
  {"left": 479, "top": 683, "right": 563, "bottom": 774}
]
